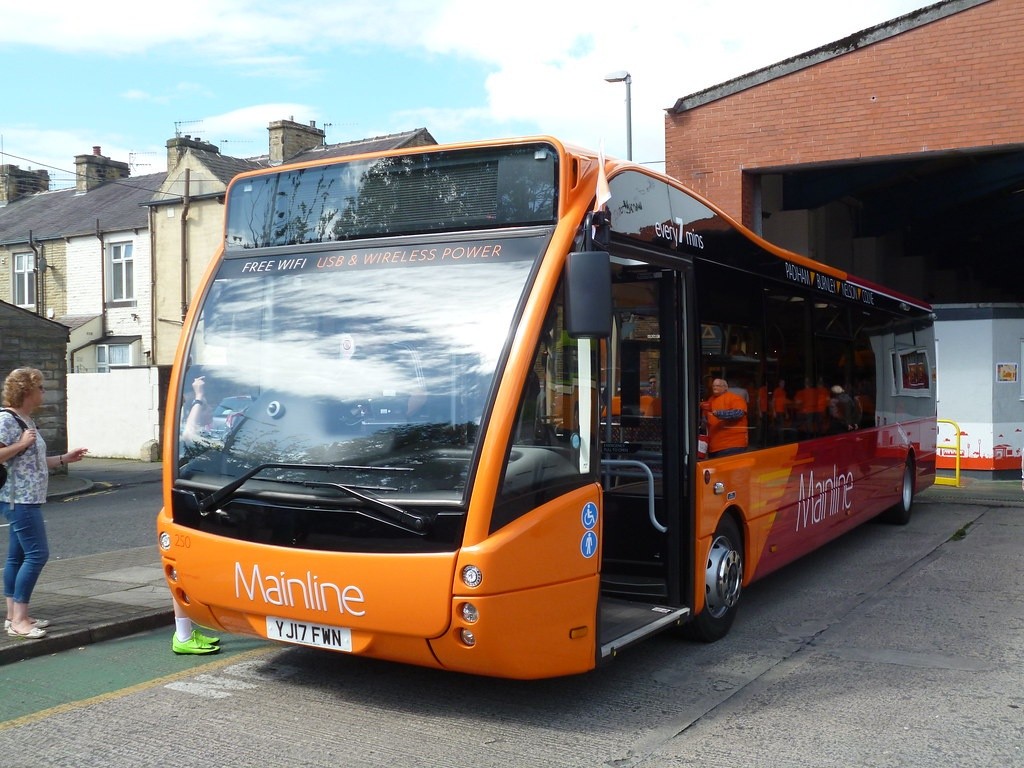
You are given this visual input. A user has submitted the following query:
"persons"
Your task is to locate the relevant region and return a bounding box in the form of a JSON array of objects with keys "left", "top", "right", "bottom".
[
  {"left": 169, "top": 376, "right": 221, "bottom": 655},
  {"left": 644, "top": 375, "right": 658, "bottom": 397},
  {"left": 340, "top": 316, "right": 428, "bottom": 416},
  {"left": 756, "top": 379, "right": 831, "bottom": 449},
  {"left": 0, "top": 364, "right": 90, "bottom": 637},
  {"left": 703, "top": 379, "right": 750, "bottom": 462}
]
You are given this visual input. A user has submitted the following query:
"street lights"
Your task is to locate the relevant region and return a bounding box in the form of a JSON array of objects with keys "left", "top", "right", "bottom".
[{"left": 605, "top": 70, "right": 634, "bottom": 164}]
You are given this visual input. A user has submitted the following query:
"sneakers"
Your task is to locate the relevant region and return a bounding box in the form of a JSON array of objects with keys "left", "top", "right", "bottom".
[{"left": 172, "top": 633, "right": 220, "bottom": 655}]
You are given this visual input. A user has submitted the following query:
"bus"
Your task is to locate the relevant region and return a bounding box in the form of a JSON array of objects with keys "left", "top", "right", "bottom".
[{"left": 159, "top": 137, "right": 943, "bottom": 682}]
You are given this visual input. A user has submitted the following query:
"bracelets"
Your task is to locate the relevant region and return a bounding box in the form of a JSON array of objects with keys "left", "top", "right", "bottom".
[
  {"left": 59, "top": 454, "right": 64, "bottom": 465},
  {"left": 191, "top": 399, "right": 203, "bottom": 407}
]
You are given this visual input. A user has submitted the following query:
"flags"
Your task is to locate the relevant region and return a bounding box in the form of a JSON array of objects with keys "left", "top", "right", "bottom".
[{"left": 587, "top": 151, "right": 613, "bottom": 243}]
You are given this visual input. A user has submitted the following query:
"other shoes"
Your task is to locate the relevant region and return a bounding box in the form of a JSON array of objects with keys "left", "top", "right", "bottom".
[
  {"left": 7, "top": 624, "right": 47, "bottom": 639},
  {"left": 4, "top": 617, "right": 50, "bottom": 630}
]
